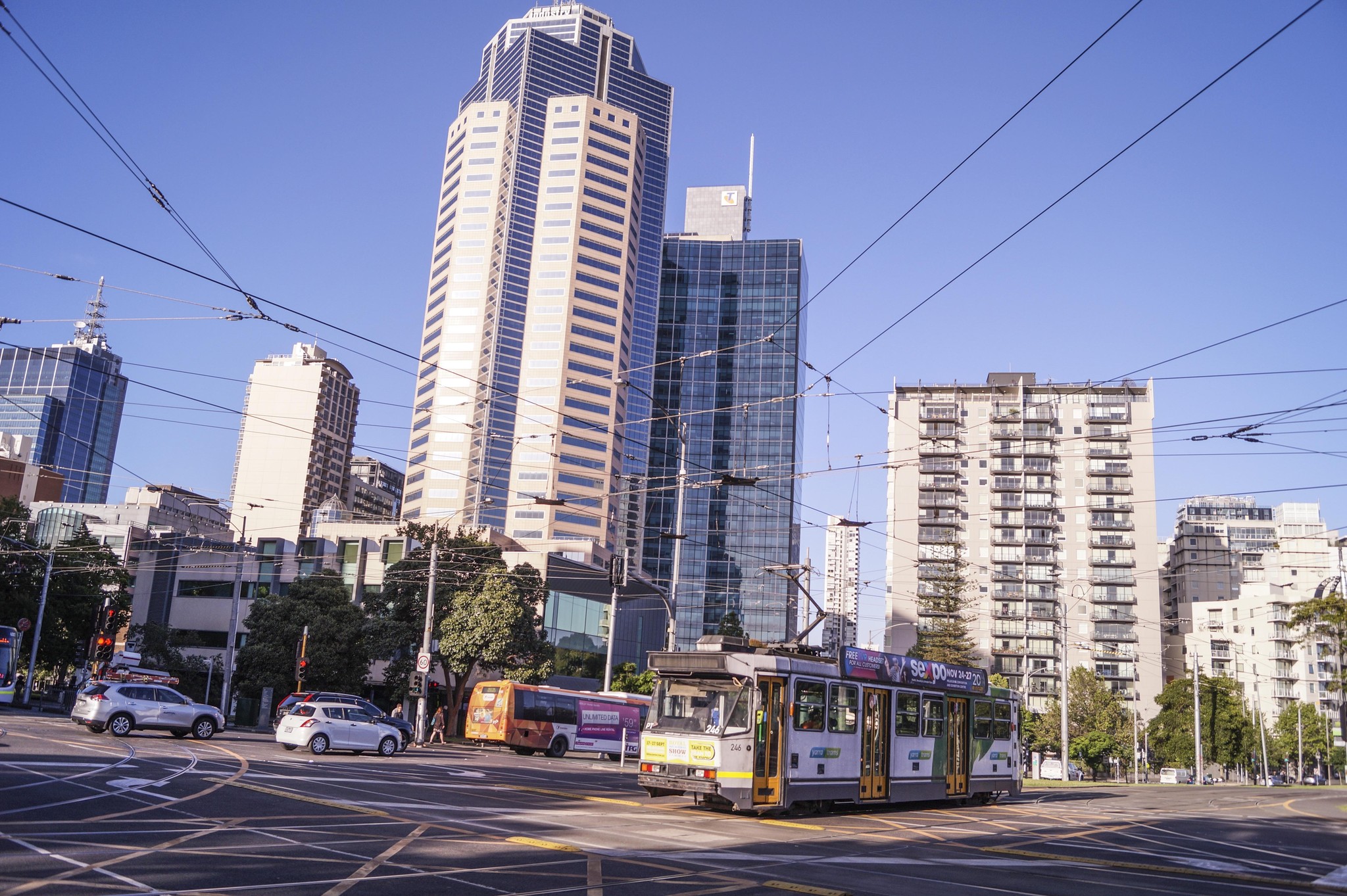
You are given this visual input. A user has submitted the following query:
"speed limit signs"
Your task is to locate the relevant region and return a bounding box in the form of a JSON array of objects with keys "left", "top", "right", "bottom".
[{"left": 418, "top": 656, "right": 429, "bottom": 669}]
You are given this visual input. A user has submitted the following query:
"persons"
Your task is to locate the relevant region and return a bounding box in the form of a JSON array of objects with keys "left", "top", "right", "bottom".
[
  {"left": 901, "top": 666, "right": 910, "bottom": 684},
  {"left": 362, "top": 698, "right": 372, "bottom": 712},
  {"left": 1280, "top": 770, "right": 1286, "bottom": 783},
  {"left": 1272, "top": 771, "right": 1277, "bottom": 776},
  {"left": 391, "top": 703, "right": 404, "bottom": 718},
  {"left": 883, "top": 655, "right": 906, "bottom": 684},
  {"left": 424, "top": 709, "right": 429, "bottom": 739},
  {"left": 15, "top": 675, "right": 25, "bottom": 702},
  {"left": 393, "top": 706, "right": 403, "bottom": 720},
  {"left": 799, "top": 708, "right": 837, "bottom": 731},
  {"left": 544, "top": 700, "right": 553, "bottom": 715},
  {"left": 76, "top": 674, "right": 98, "bottom": 697},
  {"left": 429, "top": 706, "right": 447, "bottom": 745},
  {"left": 478, "top": 712, "right": 485, "bottom": 723},
  {"left": 484, "top": 709, "right": 491, "bottom": 722}
]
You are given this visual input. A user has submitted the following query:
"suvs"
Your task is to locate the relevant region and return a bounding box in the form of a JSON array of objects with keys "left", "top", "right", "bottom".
[
  {"left": 71, "top": 680, "right": 227, "bottom": 740},
  {"left": 273, "top": 691, "right": 414, "bottom": 752}
]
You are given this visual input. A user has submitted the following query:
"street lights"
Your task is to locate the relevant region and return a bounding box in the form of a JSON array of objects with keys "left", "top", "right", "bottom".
[
  {"left": 1060, "top": 603, "right": 1090, "bottom": 782},
  {"left": 146, "top": 486, "right": 248, "bottom": 725},
  {"left": 203, "top": 656, "right": 214, "bottom": 706},
  {"left": 413, "top": 497, "right": 495, "bottom": 748}
]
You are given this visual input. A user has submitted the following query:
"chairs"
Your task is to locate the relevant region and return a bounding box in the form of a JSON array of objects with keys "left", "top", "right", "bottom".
[{"left": 538, "top": 706, "right": 547, "bottom": 721}]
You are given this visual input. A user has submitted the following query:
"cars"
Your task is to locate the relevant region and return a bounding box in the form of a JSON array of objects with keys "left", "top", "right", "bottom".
[
  {"left": 1303, "top": 774, "right": 1327, "bottom": 785},
  {"left": 1260, "top": 776, "right": 1283, "bottom": 786},
  {"left": 1187, "top": 775, "right": 1214, "bottom": 785},
  {"left": 275, "top": 701, "right": 402, "bottom": 757}
]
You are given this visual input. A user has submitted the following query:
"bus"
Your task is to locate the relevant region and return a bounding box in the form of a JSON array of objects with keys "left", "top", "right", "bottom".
[
  {"left": 0, "top": 625, "right": 20, "bottom": 703},
  {"left": 464, "top": 679, "right": 653, "bottom": 759},
  {"left": 638, "top": 634, "right": 1027, "bottom": 817}
]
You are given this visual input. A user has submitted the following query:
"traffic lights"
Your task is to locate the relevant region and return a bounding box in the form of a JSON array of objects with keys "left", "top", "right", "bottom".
[
  {"left": 409, "top": 671, "right": 425, "bottom": 696},
  {"left": 1285, "top": 755, "right": 1288, "bottom": 762},
  {"left": 295, "top": 657, "right": 310, "bottom": 682},
  {"left": 101, "top": 606, "right": 118, "bottom": 634},
  {"left": 94, "top": 634, "right": 116, "bottom": 662},
  {"left": 1120, "top": 760, "right": 1122, "bottom": 765},
  {"left": 1252, "top": 755, "right": 1255, "bottom": 761},
  {"left": 428, "top": 681, "right": 440, "bottom": 688}
]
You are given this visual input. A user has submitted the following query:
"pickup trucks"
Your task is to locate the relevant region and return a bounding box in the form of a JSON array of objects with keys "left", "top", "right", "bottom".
[{"left": 1041, "top": 759, "right": 1084, "bottom": 781}]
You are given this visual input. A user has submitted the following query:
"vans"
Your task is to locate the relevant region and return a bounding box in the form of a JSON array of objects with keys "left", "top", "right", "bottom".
[{"left": 1160, "top": 768, "right": 1189, "bottom": 784}]
